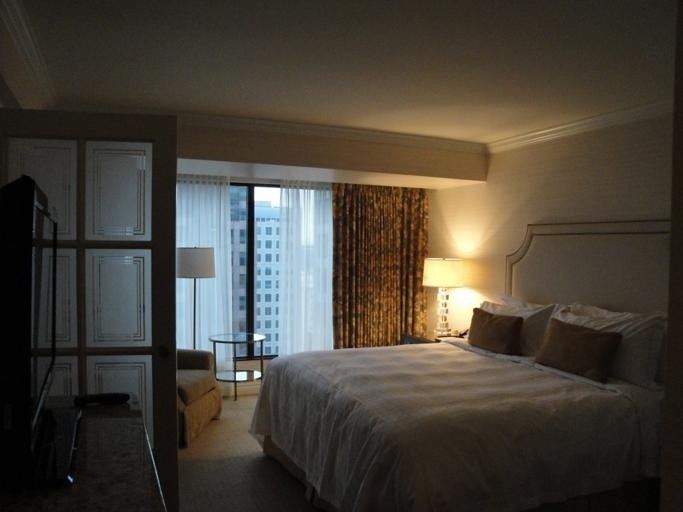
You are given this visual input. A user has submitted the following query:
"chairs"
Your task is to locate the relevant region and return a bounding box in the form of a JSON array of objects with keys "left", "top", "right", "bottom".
[{"left": 177, "top": 349, "right": 223, "bottom": 450}]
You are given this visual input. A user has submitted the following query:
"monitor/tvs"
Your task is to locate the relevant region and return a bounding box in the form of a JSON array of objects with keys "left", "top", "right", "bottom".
[{"left": 0, "top": 172, "right": 83, "bottom": 489}]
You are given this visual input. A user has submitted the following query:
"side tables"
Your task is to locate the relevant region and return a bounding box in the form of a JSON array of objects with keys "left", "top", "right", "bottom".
[{"left": 209, "top": 333, "right": 266, "bottom": 400}]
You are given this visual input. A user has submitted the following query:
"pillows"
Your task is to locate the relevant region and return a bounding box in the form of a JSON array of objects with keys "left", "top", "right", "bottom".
[{"left": 468, "top": 296, "right": 669, "bottom": 392}]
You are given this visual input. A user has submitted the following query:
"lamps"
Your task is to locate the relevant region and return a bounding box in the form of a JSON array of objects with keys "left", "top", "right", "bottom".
[
  {"left": 176, "top": 247, "right": 216, "bottom": 349},
  {"left": 423, "top": 256, "right": 474, "bottom": 338}
]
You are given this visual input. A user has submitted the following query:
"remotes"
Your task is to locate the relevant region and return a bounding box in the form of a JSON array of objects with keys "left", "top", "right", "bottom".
[{"left": 73, "top": 392, "right": 130, "bottom": 405}]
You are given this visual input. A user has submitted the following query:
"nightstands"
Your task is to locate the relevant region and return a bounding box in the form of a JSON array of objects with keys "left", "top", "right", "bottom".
[{"left": 403, "top": 332, "right": 464, "bottom": 344}]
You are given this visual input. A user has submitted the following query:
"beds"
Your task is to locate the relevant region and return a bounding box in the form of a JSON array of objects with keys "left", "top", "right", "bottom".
[{"left": 264, "top": 219, "right": 671, "bottom": 511}]
